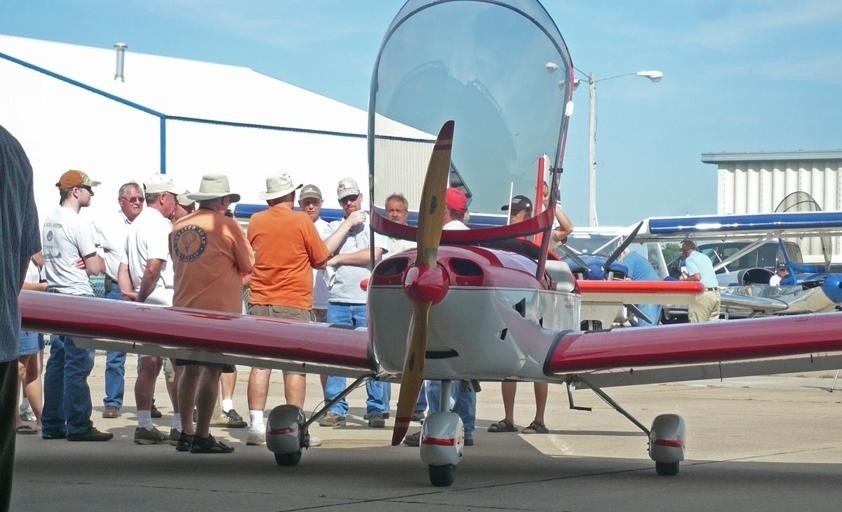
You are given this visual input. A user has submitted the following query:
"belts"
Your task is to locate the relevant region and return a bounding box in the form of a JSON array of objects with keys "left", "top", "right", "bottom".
[{"left": 703, "top": 286, "right": 719, "bottom": 292}]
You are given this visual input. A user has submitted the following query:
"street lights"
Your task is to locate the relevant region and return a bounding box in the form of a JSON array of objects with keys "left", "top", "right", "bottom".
[{"left": 546, "top": 58, "right": 662, "bottom": 230}]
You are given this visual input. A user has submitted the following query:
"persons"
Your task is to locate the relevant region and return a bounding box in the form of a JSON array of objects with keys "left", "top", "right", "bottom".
[
  {"left": 620, "top": 243, "right": 665, "bottom": 325},
  {"left": 1, "top": 127, "right": 43, "bottom": 512},
  {"left": 768, "top": 261, "right": 790, "bottom": 288},
  {"left": 488, "top": 187, "right": 572, "bottom": 433},
  {"left": 676, "top": 240, "right": 720, "bottom": 323},
  {"left": 14, "top": 170, "right": 481, "bottom": 453}
]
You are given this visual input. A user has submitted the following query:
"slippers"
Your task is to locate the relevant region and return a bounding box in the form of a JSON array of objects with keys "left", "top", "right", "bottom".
[{"left": 15, "top": 424, "right": 38, "bottom": 435}]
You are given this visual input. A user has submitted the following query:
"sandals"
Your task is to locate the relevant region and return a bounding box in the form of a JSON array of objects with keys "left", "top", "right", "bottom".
[
  {"left": 521, "top": 419, "right": 551, "bottom": 434},
  {"left": 488, "top": 417, "right": 520, "bottom": 434}
]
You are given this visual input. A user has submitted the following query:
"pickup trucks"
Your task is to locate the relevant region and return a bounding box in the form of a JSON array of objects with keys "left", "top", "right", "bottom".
[{"left": 699, "top": 241, "right": 841, "bottom": 277}]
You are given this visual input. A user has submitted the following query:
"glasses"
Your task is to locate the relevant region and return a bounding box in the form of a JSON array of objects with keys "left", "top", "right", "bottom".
[
  {"left": 78, "top": 184, "right": 92, "bottom": 192},
  {"left": 121, "top": 195, "right": 145, "bottom": 203},
  {"left": 339, "top": 194, "right": 360, "bottom": 203}
]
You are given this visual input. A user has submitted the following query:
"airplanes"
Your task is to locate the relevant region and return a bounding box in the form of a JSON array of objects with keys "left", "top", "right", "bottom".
[
  {"left": 17, "top": 0, "right": 842, "bottom": 485},
  {"left": 698, "top": 190, "right": 840, "bottom": 320},
  {"left": 548, "top": 223, "right": 663, "bottom": 331}
]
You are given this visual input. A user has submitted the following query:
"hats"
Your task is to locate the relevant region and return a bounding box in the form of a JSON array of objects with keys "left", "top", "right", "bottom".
[
  {"left": 335, "top": 176, "right": 361, "bottom": 202},
  {"left": 776, "top": 262, "right": 786, "bottom": 270},
  {"left": 500, "top": 194, "right": 534, "bottom": 215},
  {"left": 143, "top": 173, "right": 189, "bottom": 197},
  {"left": 175, "top": 190, "right": 200, "bottom": 207},
  {"left": 184, "top": 173, "right": 241, "bottom": 205},
  {"left": 443, "top": 187, "right": 470, "bottom": 217},
  {"left": 55, "top": 169, "right": 103, "bottom": 194},
  {"left": 299, "top": 181, "right": 323, "bottom": 202},
  {"left": 260, "top": 171, "right": 305, "bottom": 202}
]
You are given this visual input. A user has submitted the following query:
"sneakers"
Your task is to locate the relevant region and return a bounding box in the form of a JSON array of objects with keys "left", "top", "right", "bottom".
[
  {"left": 188, "top": 432, "right": 235, "bottom": 454},
  {"left": 175, "top": 430, "right": 197, "bottom": 452},
  {"left": 168, "top": 427, "right": 183, "bottom": 446},
  {"left": 134, "top": 424, "right": 171, "bottom": 446},
  {"left": 207, "top": 414, "right": 249, "bottom": 430},
  {"left": 316, "top": 410, "right": 350, "bottom": 428},
  {"left": 192, "top": 408, "right": 200, "bottom": 425},
  {"left": 221, "top": 408, "right": 249, "bottom": 430},
  {"left": 364, "top": 409, "right": 392, "bottom": 429},
  {"left": 409, "top": 408, "right": 426, "bottom": 423},
  {"left": 101, "top": 404, "right": 122, "bottom": 418},
  {"left": 42, "top": 428, "right": 69, "bottom": 440},
  {"left": 458, "top": 438, "right": 475, "bottom": 446},
  {"left": 242, "top": 424, "right": 269, "bottom": 448},
  {"left": 403, "top": 429, "right": 422, "bottom": 447},
  {"left": 20, "top": 406, "right": 38, "bottom": 422},
  {"left": 67, "top": 425, "right": 115, "bottom": 442},
  {"left": 151, "top": 398, "right": 164, "bottom": 418},
  {"left": 307, "top": 431, "right": 325, "bottom": 449}
]
